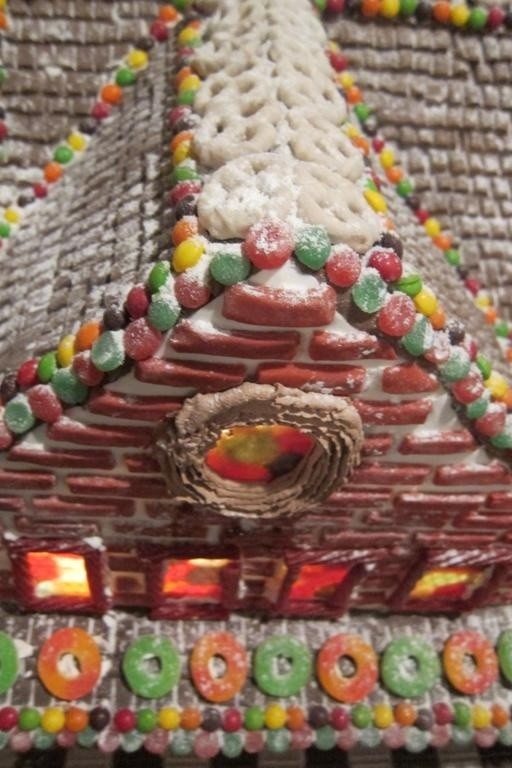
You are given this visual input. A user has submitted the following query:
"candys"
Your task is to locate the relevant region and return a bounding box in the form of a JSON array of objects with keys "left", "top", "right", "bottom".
[{"left": 1, "top": 0, "right": 512, "bottom": 758}]
